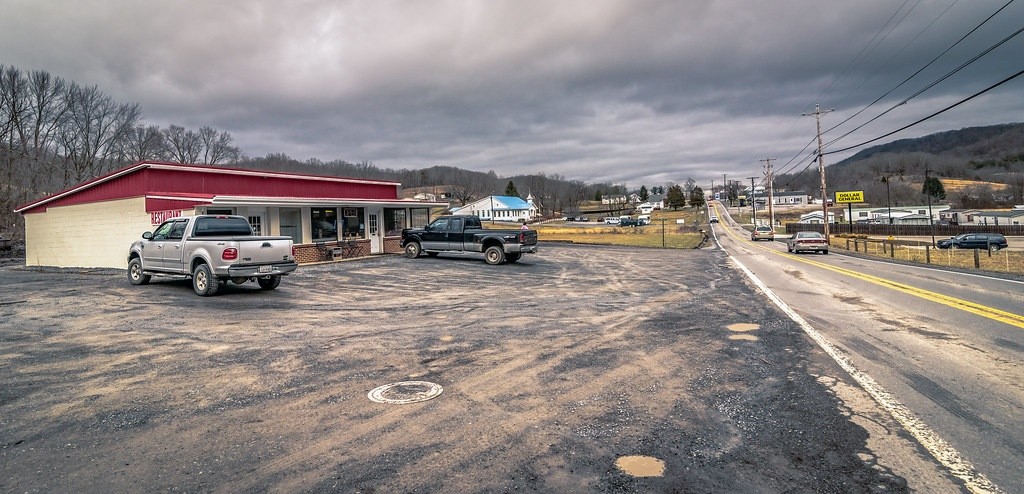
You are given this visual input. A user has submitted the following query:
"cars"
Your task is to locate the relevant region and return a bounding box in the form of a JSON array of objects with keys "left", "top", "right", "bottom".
[
  {"left": 619, "top": 215, "right": 652, "bottom": 227},
  {"left": 710, "top": 216, "right": 720, "bottom": 223},
  {"left": 786, "top": 231, "right": 829, "bottom": 254},
  {"left": 751, "top": 226, "right": 774, "bottom": 241},
  {"left": 562, "top": 216, "right": 574, "bottom": 221},
  {"left": 597, "top": 217, "right": 604, "bottom": 222},
  {"left": 582, "top": 217, "right": 590, "bottom": 221},
  {"left": 707, "top": 197, "right": 716, "bottom": 208},
  {"left": 575, "top": 217, "right": 582, "bottom": 221}
]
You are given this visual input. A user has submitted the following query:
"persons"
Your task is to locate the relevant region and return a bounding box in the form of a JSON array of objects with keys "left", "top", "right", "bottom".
[
  {"left": 521, "top": 222, "right": 528, "bottom": 230},
  {"left": 775, "top": 217, "right": 780, "bottom": 222}
]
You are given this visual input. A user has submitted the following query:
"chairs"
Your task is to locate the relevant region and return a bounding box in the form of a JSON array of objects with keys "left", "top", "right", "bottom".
[{"left": 316, "top": 240, "right": 363, "bottom": 262}]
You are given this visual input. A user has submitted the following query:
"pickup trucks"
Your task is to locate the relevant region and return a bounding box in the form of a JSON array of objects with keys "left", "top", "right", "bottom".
[
  {"left": 127, "top": 215, "right": 298, "bottom": 298},
  {"left": 397, "top": 215, "right": 539, "bottom": 266}
]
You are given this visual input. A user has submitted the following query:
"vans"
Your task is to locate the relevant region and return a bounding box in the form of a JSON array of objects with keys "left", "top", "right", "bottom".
[{"left": 605, "top": 217, "right": 620, "bottom": 224}]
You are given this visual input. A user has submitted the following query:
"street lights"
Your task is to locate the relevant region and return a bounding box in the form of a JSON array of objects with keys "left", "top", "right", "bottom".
[
  {"left": 881, "top": 174, "right": 891, "bottom": 224},
  {"left": 925, "top": 170, "right": 933, "bottom": 225}
]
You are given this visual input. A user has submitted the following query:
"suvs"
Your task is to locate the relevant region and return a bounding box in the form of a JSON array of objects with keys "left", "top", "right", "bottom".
[{"left": 936, "top": 232, "right": 1008, "bottom": 252}]
